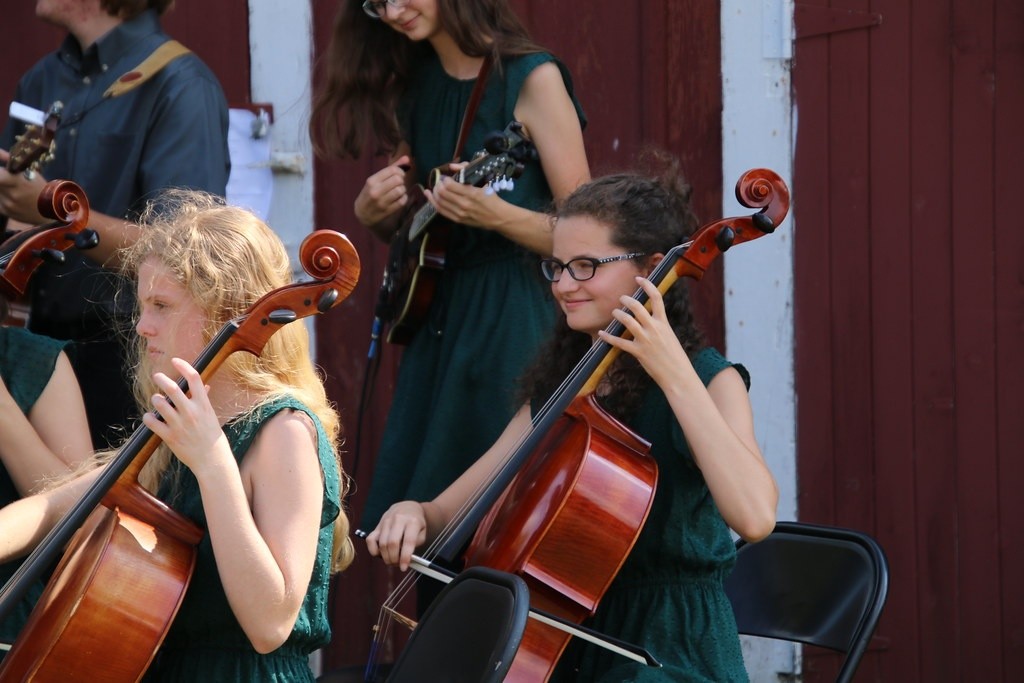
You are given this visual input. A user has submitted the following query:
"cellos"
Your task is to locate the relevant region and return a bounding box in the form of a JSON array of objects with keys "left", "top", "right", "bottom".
[
  {"left": 359, "top": 165, "right": 794, "bottom": 683},
  {"left": 1, "top": 225, "right": 362, "bottom": 683},
  {"left": 0, "top": 176, "right": 102, "bottom": 329}
]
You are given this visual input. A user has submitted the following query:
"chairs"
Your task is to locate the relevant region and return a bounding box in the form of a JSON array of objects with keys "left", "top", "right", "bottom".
[
  {"left": 729, "top": 522, "right": 889, "bottom": 683},
  {"left": 383, "top": 565, "right": 530, "bottom": 683}
]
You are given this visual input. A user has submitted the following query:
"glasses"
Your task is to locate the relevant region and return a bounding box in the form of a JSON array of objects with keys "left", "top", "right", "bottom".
[
  {"left": 540, "top": 253, "right": 647, "bottom": 282},
  {"left": 362, "top": 0, "right": 410, "bottom": 18}
]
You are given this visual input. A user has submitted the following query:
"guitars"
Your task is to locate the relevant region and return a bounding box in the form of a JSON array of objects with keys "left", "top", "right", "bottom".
[
  {"left": 383, "top": 118, "right": 529, "bottom": 348},
  {"left": 1, "top": 98, "right": 67, "bottom": 241}
]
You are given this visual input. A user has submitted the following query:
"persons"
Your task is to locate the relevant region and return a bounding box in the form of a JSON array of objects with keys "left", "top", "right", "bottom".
[
  {"left": 311, "top": 0, "right": 595, "bottom": 620},
  {"left": 0, "top": 0, "right": 230, "bottom": 461},
  {"left": 366, "top": 171, "right": 780, "bottom": 682},
  {"left": 2, "top": 206, "right": 344, "bottom": 682}
]
